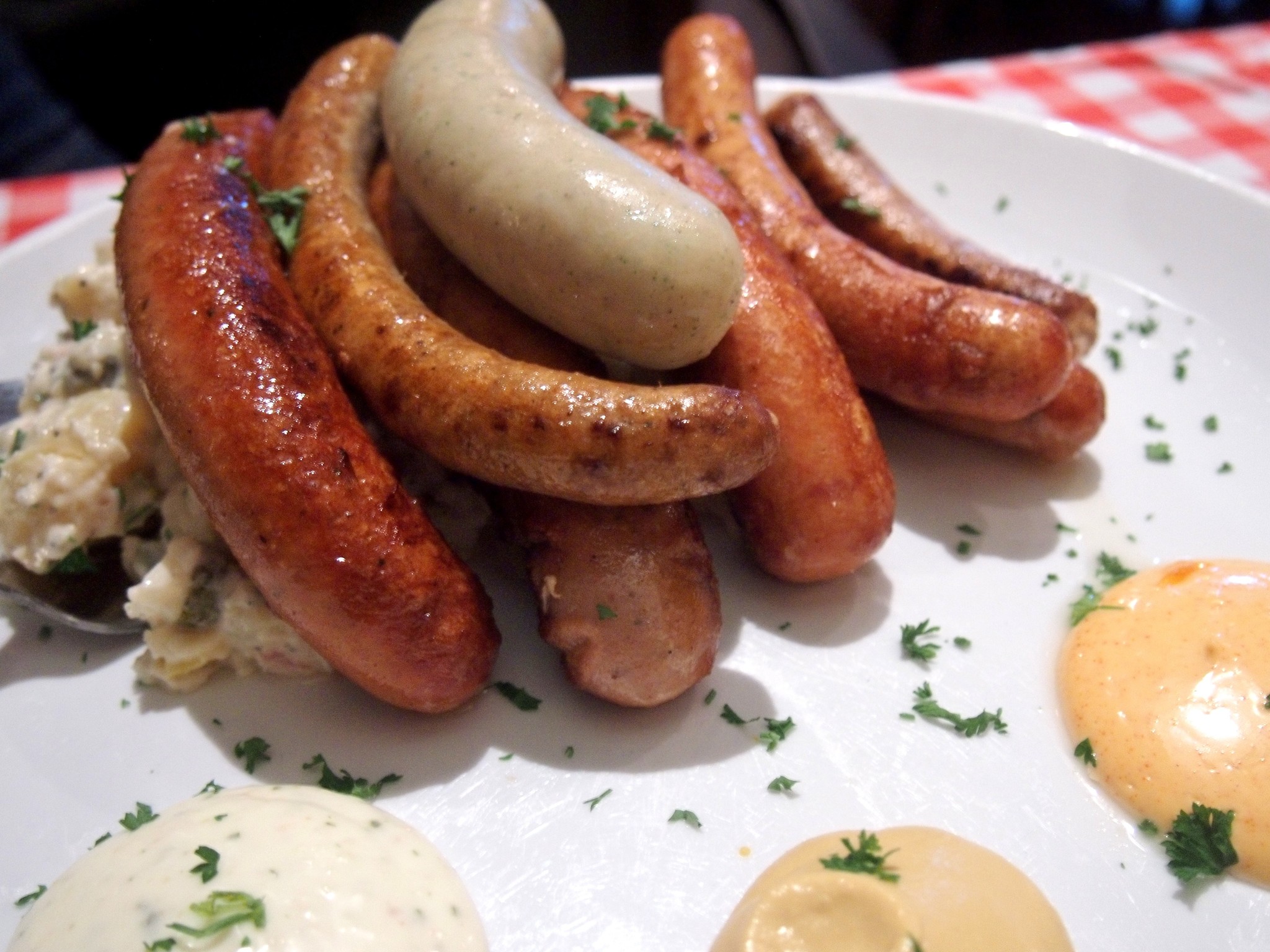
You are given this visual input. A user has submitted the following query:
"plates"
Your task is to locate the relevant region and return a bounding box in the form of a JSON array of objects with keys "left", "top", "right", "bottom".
[{"left": 0, "top": 73, "right": 1270, "bottom": 952}]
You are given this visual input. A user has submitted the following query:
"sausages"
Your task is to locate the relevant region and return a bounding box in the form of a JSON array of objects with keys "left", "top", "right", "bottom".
[{"left": 112, "top": 1, "right": 1109, "bottom": 713}]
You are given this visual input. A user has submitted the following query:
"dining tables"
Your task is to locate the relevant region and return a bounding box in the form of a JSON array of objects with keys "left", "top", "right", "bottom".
[{"left": 0, "top": 20, "right": 1270, "bottom": 240}]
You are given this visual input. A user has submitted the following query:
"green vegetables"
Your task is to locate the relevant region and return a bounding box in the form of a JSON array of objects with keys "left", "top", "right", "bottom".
[{"left": 60, "top": 102, "right": 1239, "bottom": 952}]
